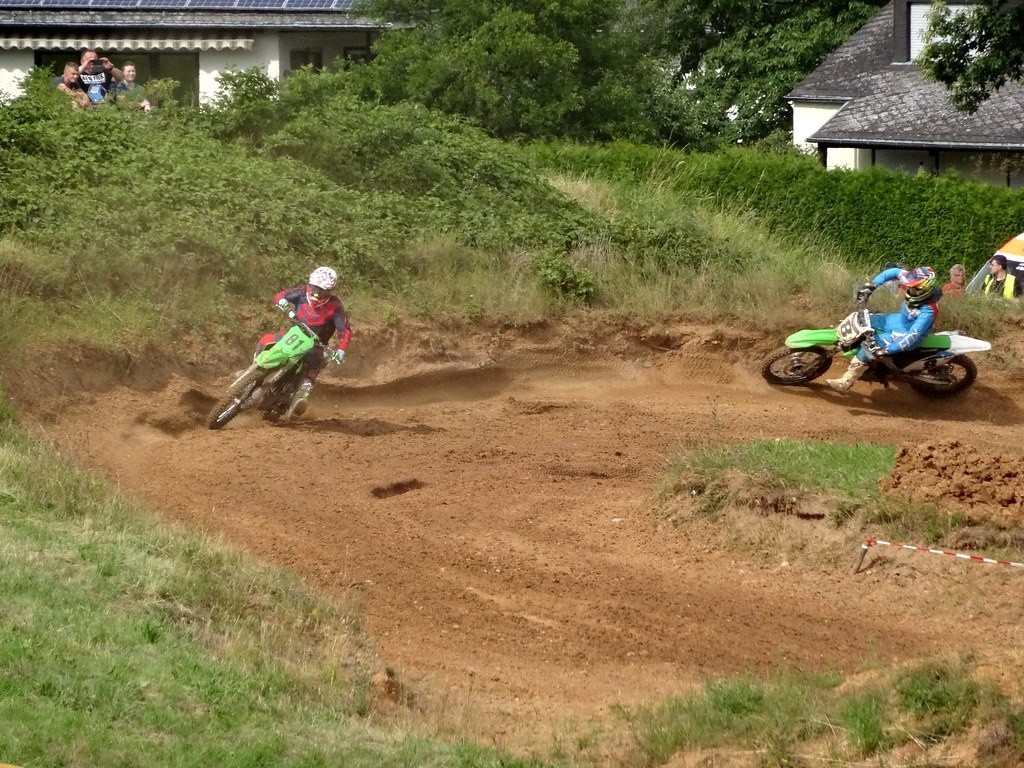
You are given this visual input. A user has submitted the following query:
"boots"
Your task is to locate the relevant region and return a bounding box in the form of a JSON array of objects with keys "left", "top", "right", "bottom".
[
  {"left": 287, "top": 377, "right": 314, "bottom": 423},
  {"left": 826, "top": 356, "right": 869, "bottom": 395},
  {"left": 235, "top": 352, "right": 256, "bottom": 378}
]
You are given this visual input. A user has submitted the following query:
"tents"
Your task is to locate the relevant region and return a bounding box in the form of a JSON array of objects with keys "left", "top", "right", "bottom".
[{"left": 967, "top": 234, "right": 1024, "bottom": 293}]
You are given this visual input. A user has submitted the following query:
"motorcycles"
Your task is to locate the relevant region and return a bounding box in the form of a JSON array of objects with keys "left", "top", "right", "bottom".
[
  {"left": 762, "top": 296, "right": 991, "bottom": 395},
  {"left": 206, "top": 303, "right": 346, "bottom": 430}
]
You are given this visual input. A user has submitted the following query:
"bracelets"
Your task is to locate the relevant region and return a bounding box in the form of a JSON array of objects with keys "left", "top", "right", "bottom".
[{"left": 108, "top": 64, "right": 114, "bottom": 71}]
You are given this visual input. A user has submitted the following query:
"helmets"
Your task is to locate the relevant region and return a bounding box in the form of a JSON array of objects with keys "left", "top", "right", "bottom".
[
  {"left": 901, "top": 266, "right": 937, "bottom": 303},
  {"left": 306, "top": 266, "right": 337, "bottom": 308}
]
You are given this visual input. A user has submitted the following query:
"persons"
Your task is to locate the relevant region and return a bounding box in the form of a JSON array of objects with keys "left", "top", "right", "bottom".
[
  {"left": 826, "top": 267, "right": 943, "bottom": 392},
  {"left": 980, "top": 255, "right": 1023, "bottom": 305},
  {"left": 228, "top": 266, "right": 352, "bottom": 421},
  {"left": 116, "top": 62, "right": 151, "bottom": 111},
  {"left": 55, "top": 62, "right": 92, "bottom": 111},
  {"left": 943, "top": 265, "right": 966, "bottom": 299},
  {"left": 78, "top": 50, "right": 124, "bottom": 109}
]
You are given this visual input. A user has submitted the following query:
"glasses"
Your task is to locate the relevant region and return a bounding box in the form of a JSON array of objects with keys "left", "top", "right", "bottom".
[{"left": 907, "top": 285, "right": 925, "bottom": 297}]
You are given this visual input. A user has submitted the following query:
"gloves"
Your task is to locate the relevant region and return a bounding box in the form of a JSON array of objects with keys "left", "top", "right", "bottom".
[
  {"left": 857, "top": 284, "right": 874, "bottom": 303},
  {"left": 868, "top": 342, "right": 886, "bottom": 357},
  {"left": 279, "top": 297, "right": 287, "bottom": 308},
  {"left": 332, "top": 349, "right": 344, "bottom": 364}
]
